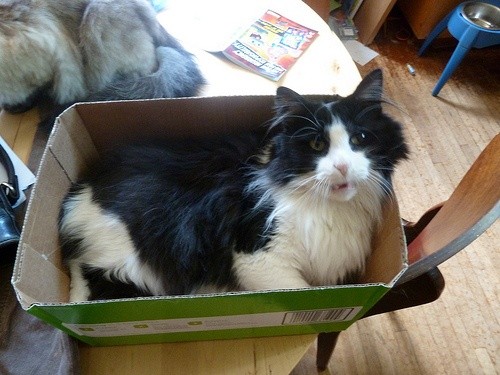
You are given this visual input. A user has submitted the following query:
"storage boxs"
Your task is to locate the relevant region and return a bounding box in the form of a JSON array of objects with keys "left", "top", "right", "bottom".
[{"left": 10, "top": 94, "right": 409, "bottom": 346}]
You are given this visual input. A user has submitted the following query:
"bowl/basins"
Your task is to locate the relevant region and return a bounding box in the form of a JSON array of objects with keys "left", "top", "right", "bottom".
[{"left": 462, "top": 2, "right": 500, "bottom": 31}]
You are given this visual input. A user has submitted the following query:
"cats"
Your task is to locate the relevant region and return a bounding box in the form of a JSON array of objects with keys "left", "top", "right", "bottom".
[
  {"left": 0, "top": 0, "right": 210, "bottom": 115},
  {"left": 55, "top": 68, "right": 415, "bottom": 305}
]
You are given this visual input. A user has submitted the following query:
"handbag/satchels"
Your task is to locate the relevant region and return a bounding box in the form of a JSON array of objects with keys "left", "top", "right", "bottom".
[{"left": 0, "top": 143, "right": 21, "bottom": 255}]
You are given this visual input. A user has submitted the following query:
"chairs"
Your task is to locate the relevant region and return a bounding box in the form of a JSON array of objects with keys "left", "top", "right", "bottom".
[{"left": 316, "top": 132, "right": 500, "bottom": 371}]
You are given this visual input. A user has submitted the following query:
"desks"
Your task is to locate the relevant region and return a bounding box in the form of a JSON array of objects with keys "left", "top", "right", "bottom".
[{"left": 0, "top": 0, "right": 374, "bottom": 375}]
